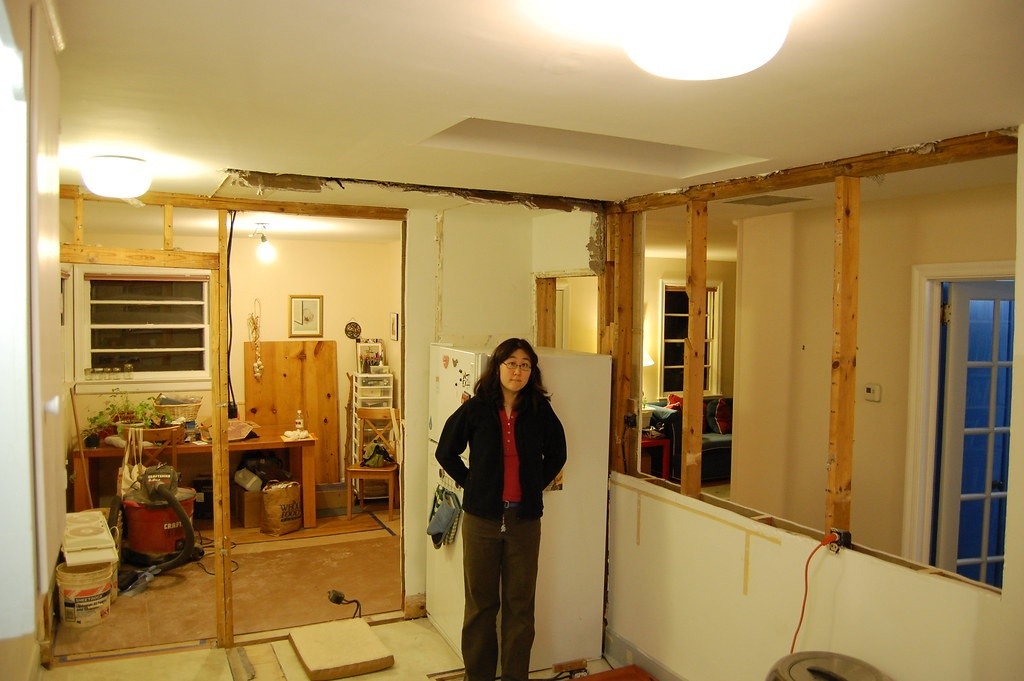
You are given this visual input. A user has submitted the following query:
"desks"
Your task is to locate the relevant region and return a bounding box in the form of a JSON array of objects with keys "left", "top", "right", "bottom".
[{"left": 74, "top": 423, "right": 318, "bottom": 530}]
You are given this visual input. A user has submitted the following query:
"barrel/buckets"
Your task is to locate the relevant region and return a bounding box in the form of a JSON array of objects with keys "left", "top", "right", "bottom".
[
  {"left": 55, "top": 561, "right": 112, "bottom": 627},
  {"left": 110, "top": 548, "right": 118, "bottom": 606},
  {"left": 190, "top": 473, "right": 213, "bottom": 522},
  {"left": 122, "top": 486, "right": 197, "bottom": 556}
]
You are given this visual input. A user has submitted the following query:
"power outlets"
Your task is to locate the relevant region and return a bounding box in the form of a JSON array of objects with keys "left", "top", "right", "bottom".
[{"left": 829, "top": 528, "right": 852, "bottom": 554}]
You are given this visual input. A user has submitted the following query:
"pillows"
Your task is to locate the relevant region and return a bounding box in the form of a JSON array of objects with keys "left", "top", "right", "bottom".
[
  {"left": 708, "top": 398, "right": 732, "bottom": 435},
  {"left": 666, "top": 394, "right": 706, "bottom": 434}
]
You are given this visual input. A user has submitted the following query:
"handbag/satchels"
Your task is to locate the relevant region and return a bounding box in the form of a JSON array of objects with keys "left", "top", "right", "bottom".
[
  {"left": 116, "top": 464, "right": 148, "bottom": 499},
  {"left": 259, "top": 480, "right": 303, "bottom": 537}
]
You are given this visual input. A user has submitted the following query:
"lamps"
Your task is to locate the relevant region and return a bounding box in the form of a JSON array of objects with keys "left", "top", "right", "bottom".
[
  {"left": 82, "top": 154, "right": 154, "bottom": 199},
  {"left": 250, "top": 230, "right": 277, "bottom": 264}
]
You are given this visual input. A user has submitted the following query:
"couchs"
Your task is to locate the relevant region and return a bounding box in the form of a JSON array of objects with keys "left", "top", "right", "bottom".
[{"left": 648, "top": 398, "right": 732, "bottom": 484}]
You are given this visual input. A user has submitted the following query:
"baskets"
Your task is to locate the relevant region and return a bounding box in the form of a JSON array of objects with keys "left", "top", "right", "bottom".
[{"left": 152, "top": 397, "right": 204, "bottom": 421}]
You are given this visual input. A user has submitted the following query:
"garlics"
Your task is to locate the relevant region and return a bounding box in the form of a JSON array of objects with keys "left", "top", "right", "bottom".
[{"left": 253, "top": 361, "right": 264, "bottom": 377}]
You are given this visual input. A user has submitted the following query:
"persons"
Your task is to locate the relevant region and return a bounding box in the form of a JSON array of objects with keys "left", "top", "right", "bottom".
[{"left": 434, "top": 338, "right": 568, "bottom": 681}]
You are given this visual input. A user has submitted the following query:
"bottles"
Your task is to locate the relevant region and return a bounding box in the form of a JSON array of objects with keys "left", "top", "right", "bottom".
[{"left": 295, "top": 410, "right": 304, "bottom": 432}]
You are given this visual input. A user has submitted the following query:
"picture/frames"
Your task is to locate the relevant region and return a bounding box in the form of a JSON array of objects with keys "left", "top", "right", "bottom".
[
  {"left": 356, "top": 342, "right": 382, "bottom": 375},
  {"left": 390, "top": 313, "right": 398, "bottom": 341},
  {"left": 288, "top": 293, "right": 323, "bottom": 338}
]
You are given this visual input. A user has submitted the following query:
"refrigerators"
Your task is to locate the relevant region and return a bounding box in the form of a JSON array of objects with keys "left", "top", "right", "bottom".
[{"left": 425, "top": 342, "right": 613, "bottom": 673}]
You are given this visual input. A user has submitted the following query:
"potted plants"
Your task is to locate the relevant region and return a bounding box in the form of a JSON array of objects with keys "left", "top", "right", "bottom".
[
  {"left": 82, "top": 386, "right": 173, "bottom": 442},
  {"left": 642, "top": 397, "right": 655, "bottom": 429}
]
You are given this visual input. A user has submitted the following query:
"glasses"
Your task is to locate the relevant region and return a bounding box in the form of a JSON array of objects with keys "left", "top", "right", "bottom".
[{"left": 501, "top": 360, "right": 532, "bottom": 371}]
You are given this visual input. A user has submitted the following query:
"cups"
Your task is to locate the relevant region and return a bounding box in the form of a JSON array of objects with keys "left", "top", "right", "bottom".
[
  {"left": 113, "top": 367, "right": 121, "bottom": 380},
  {"left": 124, "top": 364, "right": 133, "bottom": 380},
  {"left": 84, "top": 368, "right": 94, "bottom": 380},
  {"left": 94, "top": 368, "right": 103, "bottom": 380},
  {"left": 104, "top": 368, "right": 112, "bottom": 380}
]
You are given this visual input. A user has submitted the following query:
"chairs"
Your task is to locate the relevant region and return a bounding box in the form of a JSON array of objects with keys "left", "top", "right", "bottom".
[{"left": 347, "top": 406, "right": 400, "bottom": 521}]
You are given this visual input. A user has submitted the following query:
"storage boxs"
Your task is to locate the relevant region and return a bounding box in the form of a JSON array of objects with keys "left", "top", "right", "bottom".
[
  {"left": 370, "top": 366, "right": 389, "bottom": 375},
  {"left": 232, "top": 481, "right": 264, "bottom": 529}
]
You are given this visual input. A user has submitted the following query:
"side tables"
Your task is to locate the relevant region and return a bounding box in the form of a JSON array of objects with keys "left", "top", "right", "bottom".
[{"left": 640, "top": 437, "right": 672, "bottom": 480}]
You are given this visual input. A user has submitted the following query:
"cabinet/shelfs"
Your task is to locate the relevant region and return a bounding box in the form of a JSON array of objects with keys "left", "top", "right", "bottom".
[{"left": 353, "top": 373, "right": 394, "bottom": 500}]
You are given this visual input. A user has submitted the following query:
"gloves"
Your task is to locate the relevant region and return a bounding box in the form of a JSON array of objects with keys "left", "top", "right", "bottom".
[{"left": 427, "top": 490, "right": 461, "bottom": 549}]
